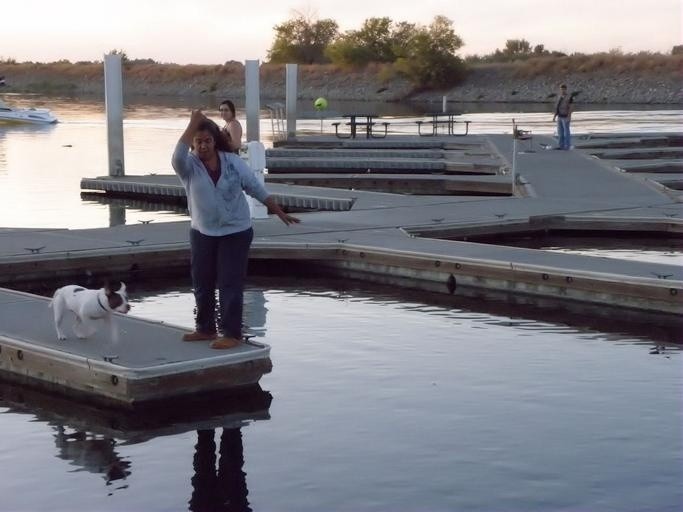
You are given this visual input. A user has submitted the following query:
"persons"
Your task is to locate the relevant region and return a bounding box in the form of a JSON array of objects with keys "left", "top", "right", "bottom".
[
  {"left": 170, "top": 100, "right": 302, "bottom": 350},
  {"left": 189, "top": 427, "right": 253, "bottom": 512},
  {"left": 552, "top": 83, "right": 576, "bottom": 151}
]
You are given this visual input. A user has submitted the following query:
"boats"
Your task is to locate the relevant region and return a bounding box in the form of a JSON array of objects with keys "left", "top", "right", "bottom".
[{"left": 0, "top": 98, "right": 59, "bottom": 124}]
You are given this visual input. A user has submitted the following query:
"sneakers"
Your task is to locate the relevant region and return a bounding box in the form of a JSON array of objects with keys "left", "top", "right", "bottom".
[
  {"left": 183, "top": 331, "right": 216, "bottom": 341},
  {"left": 209, "top": 337, "right": 241, "bottom": 349}
]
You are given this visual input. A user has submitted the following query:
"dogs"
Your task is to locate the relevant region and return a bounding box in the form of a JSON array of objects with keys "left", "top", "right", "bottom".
[{"left": 47, "top": 281, "right": 132, "bottom": 345}]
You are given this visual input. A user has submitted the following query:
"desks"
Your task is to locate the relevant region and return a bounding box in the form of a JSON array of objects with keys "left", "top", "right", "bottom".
[
  {"left": 343, "top": 112, "right": 379, "bottom": 118},
  {"left": 428, "top": 111, "right": 462, "bottom": 117},
  {"left": 513, "top": 119, "right": 534, "bottom": 154}
]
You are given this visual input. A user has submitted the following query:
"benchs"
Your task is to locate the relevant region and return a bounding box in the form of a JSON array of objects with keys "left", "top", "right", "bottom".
[
  {"left": 346, "top": 122, "right": 376, "bottom": 131},
  {"left": 464, "top": 120, "right": 471, "bottom": 135},
  {"left": 331, "top": 122, "right": 341, "bottom": 138},
  {"left": 429, "top": 120, "right": 456, "bottom": 126},
  {"left": 416, "top": 121, "right": 425, "bottom": 136},
  {"left": 378, "top": 122, "right": 390, "bottom": 137}
]
